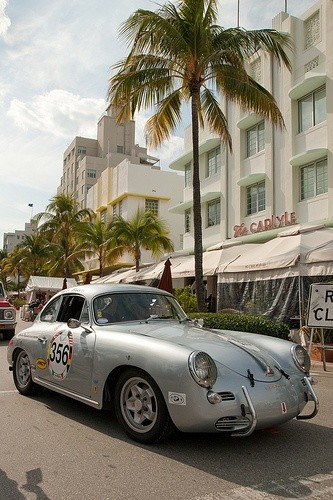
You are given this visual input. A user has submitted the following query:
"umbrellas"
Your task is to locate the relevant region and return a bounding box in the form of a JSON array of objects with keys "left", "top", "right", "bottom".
[
  {"left": 158, "top": 258, "right": 173, "bottom": 294},
  {"left": 85, "top": 272, "right": 91, "bottom": 284},
  {"left": 63, "top": 277, "right": 67, "bottom": 290}
]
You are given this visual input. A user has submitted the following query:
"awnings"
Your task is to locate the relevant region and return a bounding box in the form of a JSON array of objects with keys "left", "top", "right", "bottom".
[
  {"left": 216, "top": 224, "right": 333, "bottom": 332},
  {"left": 107, "top": 261, "right": 155, "bottom": 284},
  {"left": 172, "top": 240, "right": 265, "bottom": 284},
  {"left": 122, "top": 251, "right": 195, "bottom": 285},
  {"left": 89, "top": 267, "right": 129, "bottom": 283}
]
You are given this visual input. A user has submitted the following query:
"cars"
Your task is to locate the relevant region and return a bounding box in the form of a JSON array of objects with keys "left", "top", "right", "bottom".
[
  {"left": 7, "top": 284, "right": 320, "bottom": 444},
  {"left": 0, "top": 281, "right": 17, "bottom": 341}
]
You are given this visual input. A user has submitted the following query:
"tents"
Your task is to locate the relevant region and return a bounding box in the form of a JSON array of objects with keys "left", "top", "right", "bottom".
[{"left": 26, "top": 276, "right": 80, "bottom": 306}]
